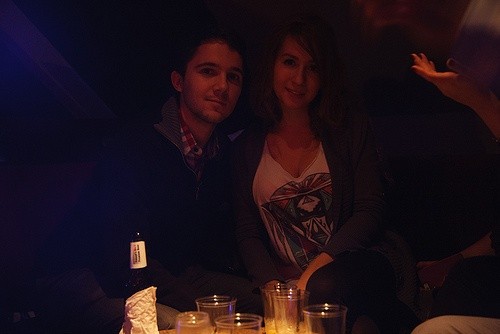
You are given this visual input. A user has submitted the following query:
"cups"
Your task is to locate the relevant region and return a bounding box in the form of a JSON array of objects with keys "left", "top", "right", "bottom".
[
  {"left": 195, "top": 295, "right": 237, "bottom": 334},
  {"left": 215, "top": 314, "right": 262, "bottom": 334},
  {"left": 176, "top": 311, "right": 210, "bottom": 334},
  {"left": 261, "top": 284, "right": 297, "bottom": 334},
  {"left": 302, "top": 304, "right": 347, "bottom": 334},
  {"left": 272, "top": 291, "right": 310, "bottom": 334}
]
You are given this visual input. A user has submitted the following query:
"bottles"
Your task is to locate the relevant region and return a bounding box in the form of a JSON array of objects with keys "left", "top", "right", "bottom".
[{"left": 124, "top": 231, "right": 156, "bottom": 334}]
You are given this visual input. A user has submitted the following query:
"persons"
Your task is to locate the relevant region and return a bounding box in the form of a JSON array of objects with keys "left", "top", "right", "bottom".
[
  {"left": 408, "top": 52, "right": 500, "bottom": 334},
  {"left": 44, "top": 24, "right": 418, "bottom": 334}
]
